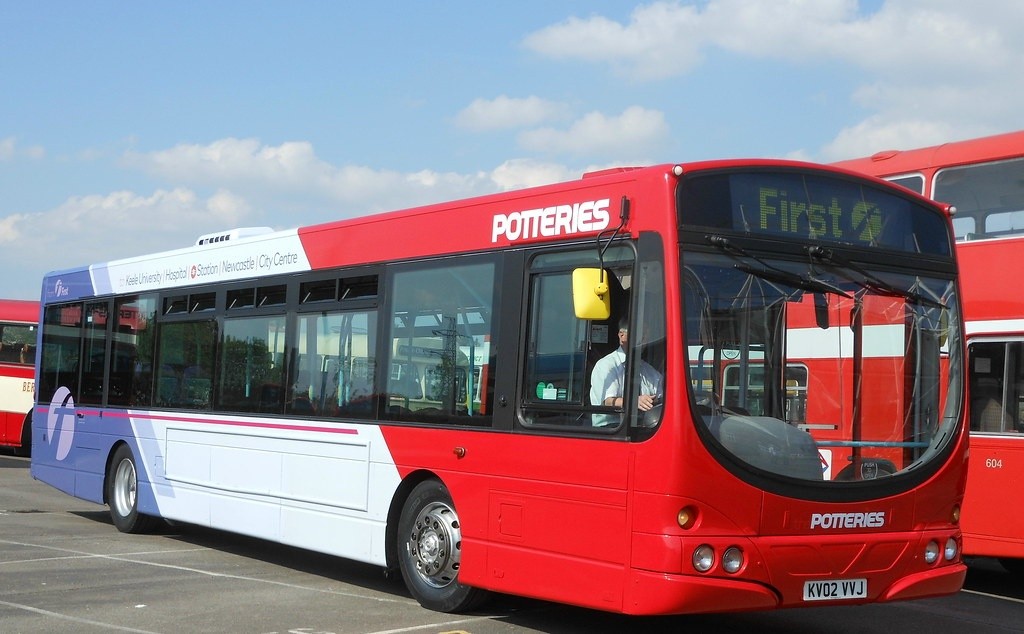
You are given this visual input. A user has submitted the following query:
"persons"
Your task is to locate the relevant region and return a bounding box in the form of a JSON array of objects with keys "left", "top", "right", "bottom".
[{"left": 590, "top": 312, "right": 663, "bottom": 428}]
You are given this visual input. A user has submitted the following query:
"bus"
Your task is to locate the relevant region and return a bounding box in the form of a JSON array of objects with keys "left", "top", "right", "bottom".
[
  {"left": 0, "top": 299, "right": 145, "bottom": 453},
  {"left": 686, "top": 132, "right": 1024, "bottom": 562},
  {"left": 30, "top": 159, "right": 967, "bottom": 619},
  {"left": 269, "top": 334, "right": 469, "bottom": 411}
]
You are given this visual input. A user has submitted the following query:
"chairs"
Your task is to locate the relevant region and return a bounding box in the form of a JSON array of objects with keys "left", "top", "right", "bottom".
[
  {"left": 137, "top": 355, "right": 338, "bottom": 413},
  {"left": 965, "top": 231, "right": 997, "bottom": 241},
  {"left": 19, "top": 343, "right": 36, "bottom": 364},
  {"left": 0, "top": 343, "right": 12, "bottom": 362}
]
